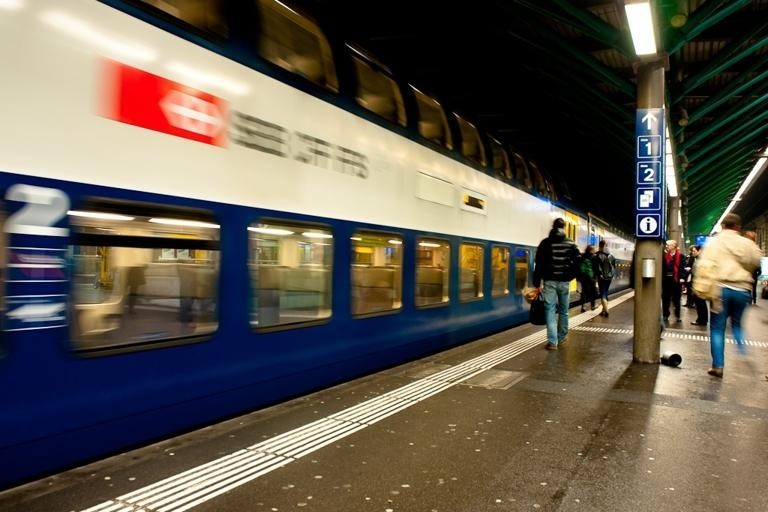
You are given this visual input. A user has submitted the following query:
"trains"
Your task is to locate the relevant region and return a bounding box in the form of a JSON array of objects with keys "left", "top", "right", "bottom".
[{"left": 0, "top": 0, "right": 637, "bottom": 492}]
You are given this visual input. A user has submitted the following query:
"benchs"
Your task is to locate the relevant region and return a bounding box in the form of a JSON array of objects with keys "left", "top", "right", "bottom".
[
  {"left": 493, "top": 262, "right": 506, "bottom": 291},
  {"left": 516, "top": 266, "right": 527, "bottom": 291},
  {"left": 251, "top": 259, "right": 332, "bottom": 321},
  {"left": 416, "top": 264, "right": 447, "bottom": 305},
  {"left": 72, "top": 258, "right": 218, "bottom": 341},
  {"left": 351, "top": 263, "right": 401, "bottom": 312},
  {"left": 460, "top": 266, "right": 479, "bottom": 301}
]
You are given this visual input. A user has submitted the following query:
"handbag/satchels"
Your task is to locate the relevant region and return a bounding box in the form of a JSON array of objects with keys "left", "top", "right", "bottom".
[{"left": 528, "top": 290, "right": 546, "bottom": 327}]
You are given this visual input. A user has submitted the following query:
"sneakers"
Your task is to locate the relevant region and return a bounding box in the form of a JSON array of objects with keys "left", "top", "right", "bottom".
[
  {"left": 689, "top": 320, "right": 708, "bottom": 327},
  {"left": 705, "top": 367, "right": 725, "bottom": 378},
  {"left": 558, "top": 335, "right": 570, "bottom": 346},
  {"left": 543, "top": 342, "right": 559, "bottom": 350}
]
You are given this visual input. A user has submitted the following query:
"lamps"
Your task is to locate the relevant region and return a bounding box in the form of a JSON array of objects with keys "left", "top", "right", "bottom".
[
  {"left": 680, "top": 178, "right": 690, "bottom": 237},
  {"left": 676, "top": 106, "right": 691, "bottom": 128},
  {"left": 623, "top": 0, "right": 661, "bottom": 59},
  {"left": 664, "top": 1, "right": 691, "bottom": 29},
  {"left": 665, "top": 124, "right": 680, "bottom": 198},
  {"left": 678, "top": 153, "right": 690, "bottom": 169}
]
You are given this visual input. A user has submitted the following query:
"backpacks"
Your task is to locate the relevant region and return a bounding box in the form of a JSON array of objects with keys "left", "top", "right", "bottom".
[{"left": 690, "top": 257, "right": 720, "bottom": 301}]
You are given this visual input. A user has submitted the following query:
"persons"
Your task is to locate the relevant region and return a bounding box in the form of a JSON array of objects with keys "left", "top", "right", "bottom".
[
  {"left": 591, "top": 240, "right": 616, "bottom": 317},
  {"left": 576, "top": 245, "right": 597, "bottom": 313},
  {"left": 532, "top": 218, "right": 580, "bottom": 350},
  {"left": 629, "top": 213, "right": 768, "bottom": 377}
]
